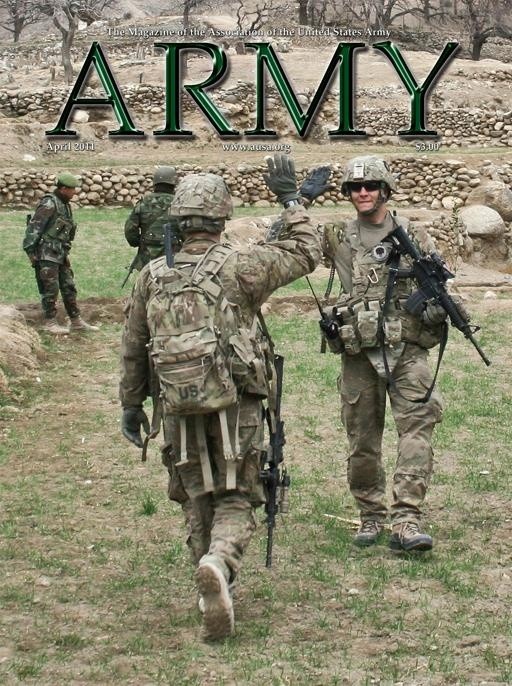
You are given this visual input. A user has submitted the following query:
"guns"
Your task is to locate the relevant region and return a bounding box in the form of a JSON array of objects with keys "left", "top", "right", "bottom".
[
  {"left": 381, "top": 226, "right": 491, "bottom": 367},
  {"left": 257, "top": 351, "right": 290, "bottom": 568}
]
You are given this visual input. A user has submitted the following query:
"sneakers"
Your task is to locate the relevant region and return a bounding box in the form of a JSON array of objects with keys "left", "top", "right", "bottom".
[
  {"left": 194, "top": 555, "right": 236, "bottom": 639},
  {"left": 389, "top": 522, "right": 433, "bottom": 551},
  {"left": 356, "top": 520, "right": 384, "bottom": 546}
]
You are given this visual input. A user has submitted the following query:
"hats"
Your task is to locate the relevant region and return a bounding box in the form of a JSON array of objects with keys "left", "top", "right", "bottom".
[{"left": 58, "top": 174, "right": 79, "bottom": 188}]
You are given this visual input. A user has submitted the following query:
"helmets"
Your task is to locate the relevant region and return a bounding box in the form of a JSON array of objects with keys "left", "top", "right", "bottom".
[
  {"left": 153, "top": 166, "right": 177, "bottom": 186},
  {"left": 168, "top": 173, "right": 232, "bottom": 220},
  {"left": 341, "top": 157, "right": 397, "bottom": 197}
]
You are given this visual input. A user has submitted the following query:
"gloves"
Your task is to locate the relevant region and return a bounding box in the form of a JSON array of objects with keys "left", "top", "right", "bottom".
[
  {"left": 422, "top": 304, "right": 447, "bottom": 326},
  {"left": 122, "top": 408, "right": 149, "bottom": 448},
  {"left": 263, "top": 154, "right": 300, "bottom": 204},
  {"left": 299, "top": 167, "right": 337, "bottom": 203}
]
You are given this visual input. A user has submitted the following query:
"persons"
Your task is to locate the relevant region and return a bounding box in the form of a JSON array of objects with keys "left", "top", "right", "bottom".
[
  {"left": 124, "top": 164, "right": 186, "bottom": 273},
  {"left": 261, "top": 153, "right": 456, "bottom": 555},
  {"left": 118, "top": 153, "right": 324, "bottom": 644},
  {"left": 21, "top": 172, "right": 100, "bottom": 337}
]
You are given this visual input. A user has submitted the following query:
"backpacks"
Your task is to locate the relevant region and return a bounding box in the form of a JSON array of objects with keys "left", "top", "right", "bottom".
[{"left": 145, "top": 243, "right": 240, "bottom": 416}]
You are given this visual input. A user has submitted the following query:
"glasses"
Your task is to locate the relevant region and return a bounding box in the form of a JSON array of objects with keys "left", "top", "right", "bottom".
[{"left": 352, "top": 182, "right": 381, "bottom": 192}]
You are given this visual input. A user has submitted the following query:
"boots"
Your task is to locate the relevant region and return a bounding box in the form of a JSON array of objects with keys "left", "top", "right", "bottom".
[
  {"left": 45, "top": 318, "right": 70, "bottom": 335},
  {"left": 71, "top": 316, "right": 100, "bottom": 332}
]
